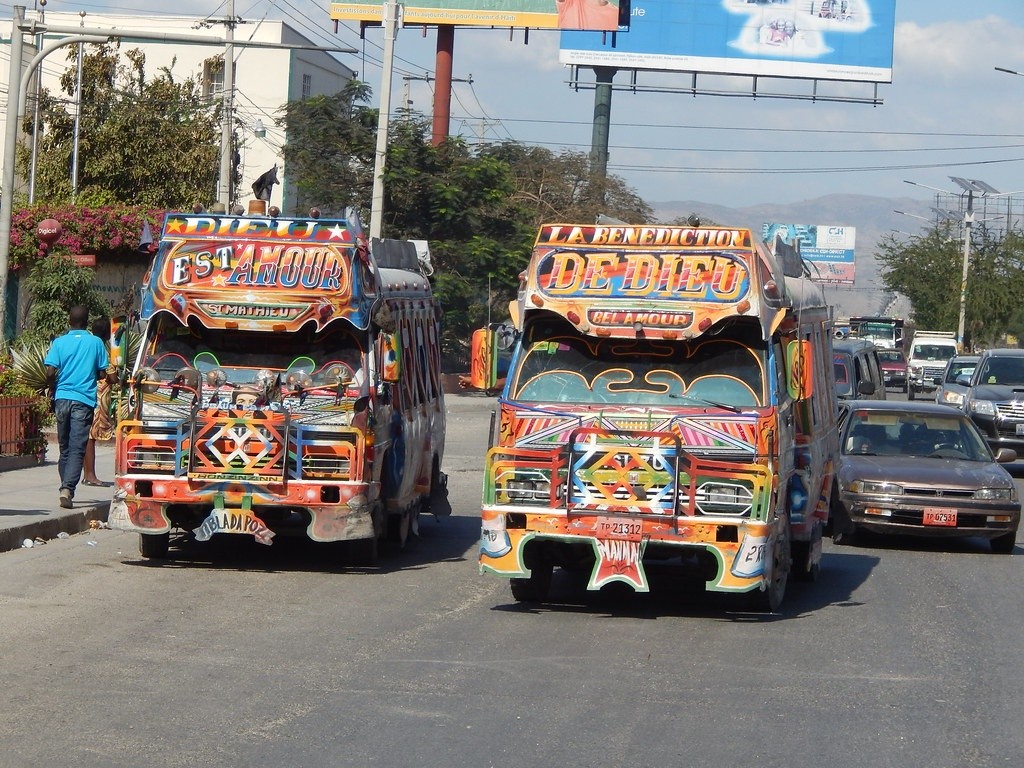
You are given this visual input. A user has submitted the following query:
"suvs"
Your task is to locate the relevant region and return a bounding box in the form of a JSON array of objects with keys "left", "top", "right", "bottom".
[
  {"left": 933, "top": 354, "right": 982, "bottom": 410},
  {"left": 903, "top": 331, "right": 958, "bottom": 400}
]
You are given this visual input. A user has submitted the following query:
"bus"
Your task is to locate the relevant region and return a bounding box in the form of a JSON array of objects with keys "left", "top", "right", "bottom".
[
  {"left": 472, "top": 224, "right": 839, "bottom": 611},
  {"left": 107, "top": 200, "right": 451, "bottom": 566}
]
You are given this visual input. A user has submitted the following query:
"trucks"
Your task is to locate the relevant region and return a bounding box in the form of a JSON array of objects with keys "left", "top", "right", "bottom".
[{"left": 847, "top": 316, "right": 904, "bottom": 348}]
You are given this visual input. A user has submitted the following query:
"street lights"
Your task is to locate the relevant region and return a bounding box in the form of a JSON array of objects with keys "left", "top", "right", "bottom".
[{"left": 891, "top": 175, "right": 1024, "bottom": 353}]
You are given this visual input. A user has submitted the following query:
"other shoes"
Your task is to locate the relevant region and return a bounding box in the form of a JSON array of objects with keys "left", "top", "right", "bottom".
[
  {"left": 82, "top": 478, "right": 90, "bottom": 484},
  {"left": 88, "top": 482, "right": 110, "bottom": 486},
  {"left": 59, "top": 488, "right": 73, "bottom": 508}
]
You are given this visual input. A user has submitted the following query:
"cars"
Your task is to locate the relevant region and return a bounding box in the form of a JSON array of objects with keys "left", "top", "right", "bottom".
[
  {"left": 959, "top": 349, "right": 1024, "bottom": 459},
  {"left": 830, "top": 400, "right": 1021, "bottom": 554},
  {"left": 876, "top": 348, "right": 909, "bottom": 393}
]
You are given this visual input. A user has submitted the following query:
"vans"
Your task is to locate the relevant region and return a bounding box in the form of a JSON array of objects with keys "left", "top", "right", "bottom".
[{"left": 832, "top": 339, "right": 886, "bottom": 401}]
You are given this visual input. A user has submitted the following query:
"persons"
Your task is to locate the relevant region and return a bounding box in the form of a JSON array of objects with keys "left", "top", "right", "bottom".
[
  {"left": 852, "top": 426, "right": 901, "bottom": 455},
  {"left": 81, "top": 315, "right": 119, "bottom": 487},
  {"left": 44, "top": 306, "right": 109, "bottom": 509},
  {"left": 903, "top": 425, "right": 959, "bottom": 453}
]
